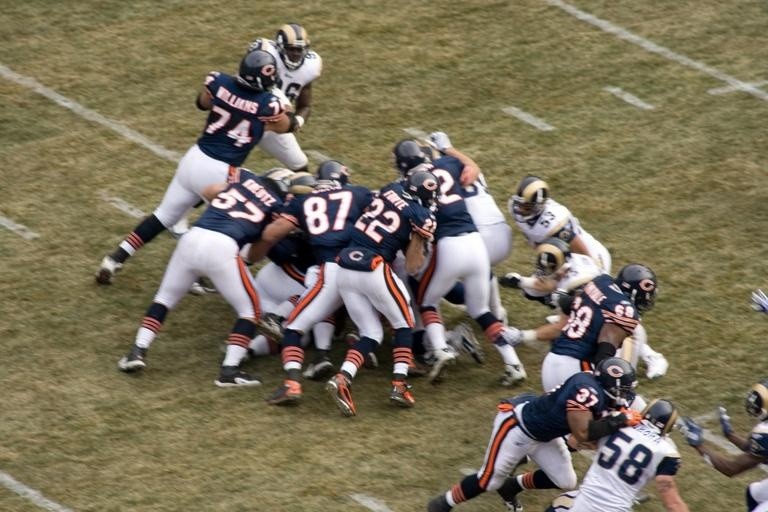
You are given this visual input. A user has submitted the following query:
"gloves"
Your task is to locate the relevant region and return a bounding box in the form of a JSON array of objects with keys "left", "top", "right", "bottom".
[
  {"left": 717, "top": 403, "right": 733, "bottom": 436},
  {"left": 499, "top": 271, "right": 521, "bottom": 289},
  {"left": 425, "top": 130, "right": 452, "bottom": 151},
  {"left": 750, "top": 288, "right": 767, "bottom": 313},
  {"left": 496, "top": 325, "right": 523, "bottom": 346},
  {"left": 677, "top": 416, "right": 704, "bottom": 449}
]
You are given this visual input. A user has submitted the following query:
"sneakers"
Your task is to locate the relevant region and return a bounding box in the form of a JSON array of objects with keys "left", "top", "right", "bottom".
[
  {"left": 497, "top": 484, "right": 523, "bottom": 512},
  {"left": 495, "top": 363, "right": 528, "bottom": 387},
  {"left": 190, "top": 283, "right": 204, "bottom": 294},
  {"left": 343, "top": 333, "right": 378, "bottom": 370},
  {"left": 214, "top": 368, "right": 263, "bottom": 388},
  {"left": 300, "top": 357, "right": 336, "bottom": 382},
  {"left": 324, "top": 373, "right": 358, "bottom": 417},
  {"left": 390, "top": 382, "right": 416, "bottom": 407},
  {"left": 92, "top": 257, "right": 121, "bottom": 288},
  {"left": 117, "top": 345, "right": 147, "bottom": 373},
  {"left": 644, "top": 353, "right": 669, "bottom": 380},
  {"left": 427, "top": 347, "right": 457, "bottom": 385},
  {"left": 265, "top": 379, "right": 306, "bottom": 405},
  {"left": 409, "top": 359, "right": 430, "bottom": 376},
  {"left": 452, "top": 319, "right": 487, "bottom": 367}
]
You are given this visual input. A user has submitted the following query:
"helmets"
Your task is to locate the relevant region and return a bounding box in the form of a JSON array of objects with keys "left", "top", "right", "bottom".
[
  {"left": 639, "top": 397, "right": 680, "bottom": 434},
  {"left": 745, "top": 381, "right": 768, "bottom": 422},
  {"left": 597, "top": 358, "right": 638, "bottom": 408},
  {"left": 318, "top": 160, "right": 350, "bottom": 185},
  {"left": 240, "top": 50, "right": 278, "bottom": 90},
  {"left": 619, "top": 261, "right": 658, "bottom": 311},
  {"left": 509, "top": 173, "right": 551, "bottom": 222},
  {"left": 535, "top": 238, "right": 564, "bottom": 274},
  {"left": 391, "top": 139, "right": 427, "bottom": 174},
  {"left": 264, "top": 166, "right": 296, "bottom": 193},
  {"left": 288, "top": 170, "right": 318, "bottom": 195},
  {"left": 403, "top": 170, "right": 441, "bottom": 207},
  {"left": 275, "top": 22, "right": 310, "bottom": 70}
]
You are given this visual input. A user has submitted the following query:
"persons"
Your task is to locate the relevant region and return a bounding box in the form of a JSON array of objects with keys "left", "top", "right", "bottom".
[
  {"left": 115, "top": 166, "right": 291, "bottom": 387},
  {"left": 687, "top": 384, "right": 768, "bottom": 511},
  {"left": 239, "top": 25, "right": 322, "bottom": 174},
  {"left": 542, "top": 263, "right": 659, "bottom": 393},
  {"left": 395, "top": 133, "right": 527, "bottom": 386},
  {"left": 507, "top": 175, "right": 613, "bottom": 272},
  {"left": 241, "top": 162, "right": 376, "bottom": 407},
  {"left": 93, "top": 51, "right": 279, "bottom": 296},
  {"left": 552, "top": 400, "right": 687, "bottom": 512},
  {"left": 498, "top": 245, "right": 669, "bottom": 381},
  {"left": 420, "top": 358, "right": 638, "bottom": 511},
  {"left": 426, "top": 131, "right": 513, "bottom": 331},
  {"left": 325, "top": 170, "right": 435, "bottom": 417}
]
